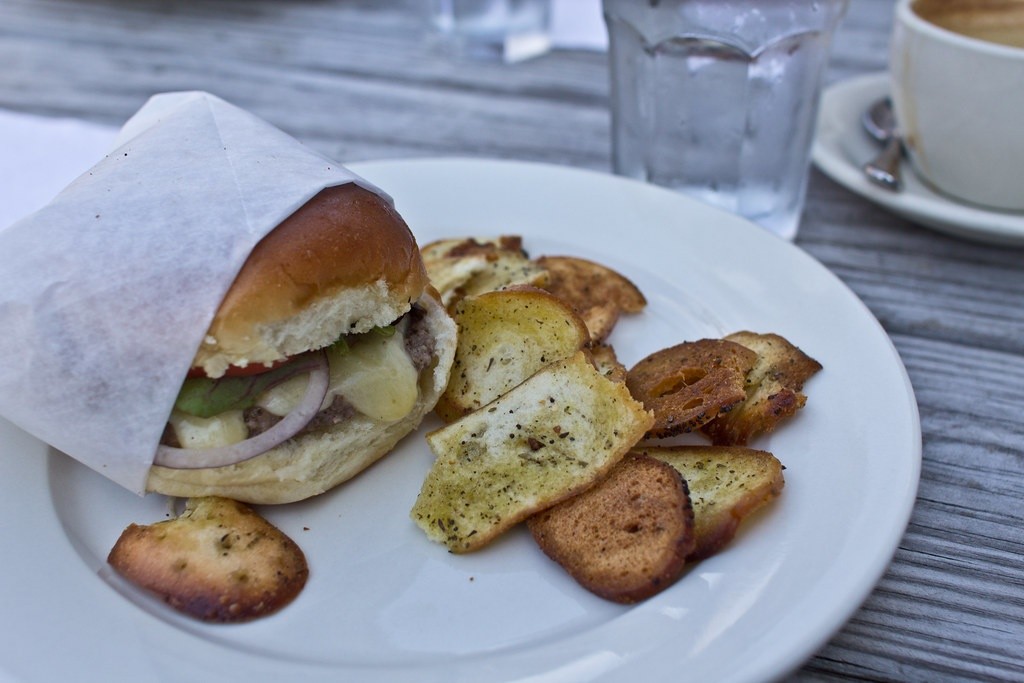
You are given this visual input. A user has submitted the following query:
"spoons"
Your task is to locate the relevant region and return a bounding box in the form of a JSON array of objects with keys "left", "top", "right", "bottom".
[{"left": 863, "top": 95, "right": 905, "bottom": 189}]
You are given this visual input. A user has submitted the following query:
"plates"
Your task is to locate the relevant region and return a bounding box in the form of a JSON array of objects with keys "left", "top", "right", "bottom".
[
  {"left": 0, "top": 157, "right": 922, "bottom": 683},
  {"left": 812, "top": 71, "right": 1024, "bottom": 247}
]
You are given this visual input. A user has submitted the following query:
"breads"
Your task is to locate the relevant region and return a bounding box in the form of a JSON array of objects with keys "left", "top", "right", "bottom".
[
  {"left": 109, "top": 495, "right": 308, "bottom": 620},
  {"left": 429, "top": 243, "right": 822, "bottom": 602}
]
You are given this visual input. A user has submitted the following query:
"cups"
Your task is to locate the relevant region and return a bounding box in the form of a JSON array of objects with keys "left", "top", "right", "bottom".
[
  {"left": 602, "top": 0, "right": 850, "bottom": 241},
  {"left": 890, "top": 0, "right": 1024, "bottom": 210},
  {"left": 428, "top": 0, "right": 551, "bottom": 64}
]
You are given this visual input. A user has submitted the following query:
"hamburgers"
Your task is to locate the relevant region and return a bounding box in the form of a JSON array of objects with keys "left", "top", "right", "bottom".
[{"left": 149, "top": 182, "right": 455, "bottom": 504}]
